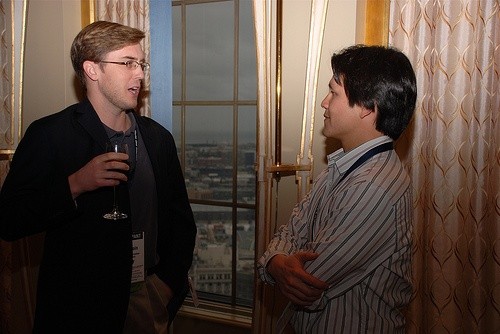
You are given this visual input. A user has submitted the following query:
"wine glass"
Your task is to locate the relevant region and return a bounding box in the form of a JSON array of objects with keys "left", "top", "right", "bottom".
[{"left": 102, "top": 128, "right": 136, "bottom": 221}]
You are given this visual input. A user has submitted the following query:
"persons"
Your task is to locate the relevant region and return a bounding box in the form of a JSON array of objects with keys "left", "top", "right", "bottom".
[
  {"left": 257, "top": 44, "right": 417, "bottom": 334},
  {"left": 0, "top": 21, "right": 197, "bottom": 334}
]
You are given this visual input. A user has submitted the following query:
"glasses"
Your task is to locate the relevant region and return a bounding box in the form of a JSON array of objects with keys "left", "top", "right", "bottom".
[{"left": 94, "top": 60, "right": 150, "bottom": 72}]
